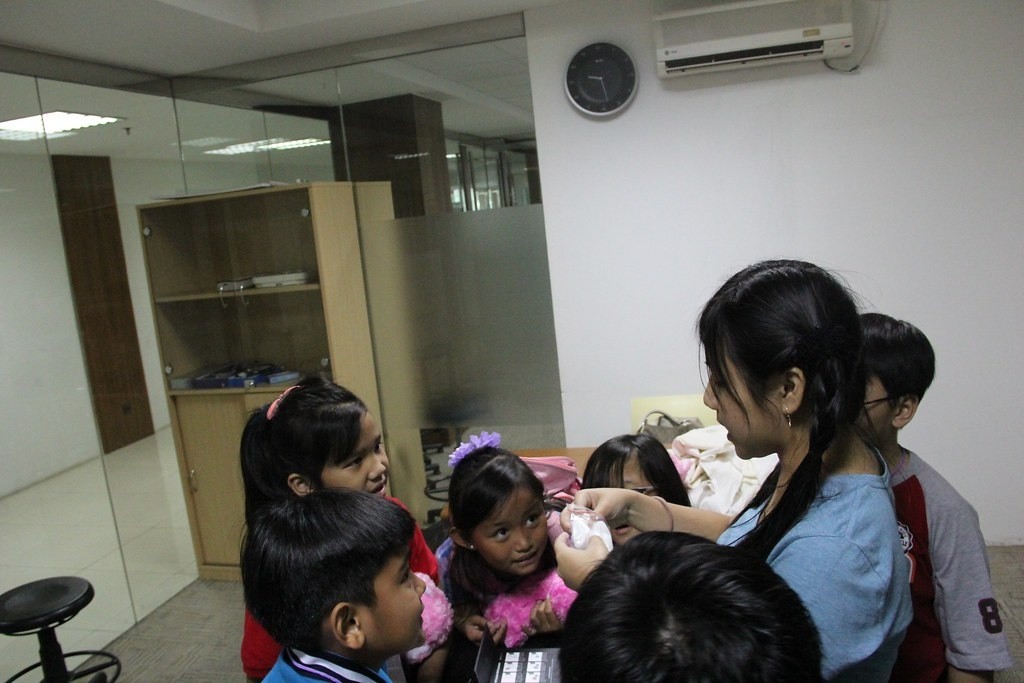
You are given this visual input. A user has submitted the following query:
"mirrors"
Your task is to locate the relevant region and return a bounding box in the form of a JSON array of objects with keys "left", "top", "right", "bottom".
[{"left": 174, "top": 34, "right": 568, "bottom": 524}]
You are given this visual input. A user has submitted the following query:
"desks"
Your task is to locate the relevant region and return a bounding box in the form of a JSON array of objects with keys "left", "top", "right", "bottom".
[{"left": 506, "top": 448, "right": 597, "bottom": 481}]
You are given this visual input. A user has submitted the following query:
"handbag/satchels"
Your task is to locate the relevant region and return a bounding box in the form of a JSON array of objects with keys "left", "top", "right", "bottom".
[{"left": 636, "top": 411, "right": 704, "bottom": 449}]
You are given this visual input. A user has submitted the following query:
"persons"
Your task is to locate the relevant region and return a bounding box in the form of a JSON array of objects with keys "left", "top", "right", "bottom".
[
  {"left": 240, "top": 488, "right": 426, "bottom": 683},
  {"left": 558, "top": 531, "right": 823, "bottom": 683},
  {"left": 435, "top": 430, "right": 561, "bottom": 643},
  {"left": 554, "top": 259, "right": 912, "bottom": 683},
  {"left": 240, "top": 375, "right": 450, "bottom": 683},
  {"left": 581, "top": 435, "right": 691, "bottom": 549},
  {"left": 860, "top": 312, "right": 1013, "bottom": 683}
]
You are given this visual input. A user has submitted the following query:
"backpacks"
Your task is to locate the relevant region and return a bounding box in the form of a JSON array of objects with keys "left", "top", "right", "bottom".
[{"left": 517, "top": 455, "right": 578, "bottom": 503}]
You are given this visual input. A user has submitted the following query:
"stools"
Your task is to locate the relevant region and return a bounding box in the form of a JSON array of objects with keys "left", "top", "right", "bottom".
[
  {"left": 421, "top": 396, "right": 485, "bottom": 503},
  {"left": 0, "top": 576, "right": 121, "bottom": 683}
]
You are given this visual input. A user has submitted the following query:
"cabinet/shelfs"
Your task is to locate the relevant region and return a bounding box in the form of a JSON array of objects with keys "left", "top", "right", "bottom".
[
  {"left": 348, "top": 177, "right": 438, "bottom": 529},
  {"left": 135, "top": 180, "right": 395, "bottom": 585}
]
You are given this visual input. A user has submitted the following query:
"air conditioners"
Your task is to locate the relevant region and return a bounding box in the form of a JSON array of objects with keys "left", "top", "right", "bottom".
[{"left": 650, "top": 0, "right": 855, "bottom": 80}]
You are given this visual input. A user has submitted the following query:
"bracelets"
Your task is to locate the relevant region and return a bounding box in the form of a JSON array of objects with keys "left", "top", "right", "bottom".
[{"left": 652, "top": 495, "right": 675, "bottom": 531}]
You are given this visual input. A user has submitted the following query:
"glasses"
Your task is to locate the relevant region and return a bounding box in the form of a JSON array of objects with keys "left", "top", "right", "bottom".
[{"left": 630, "top": 486, "right": 654, "bottom": 495}]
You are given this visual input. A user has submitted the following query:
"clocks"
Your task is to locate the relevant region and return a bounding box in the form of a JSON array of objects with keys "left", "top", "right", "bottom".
[{"left": 564, "top": 40, "right": 639, "bottom": 119}]
